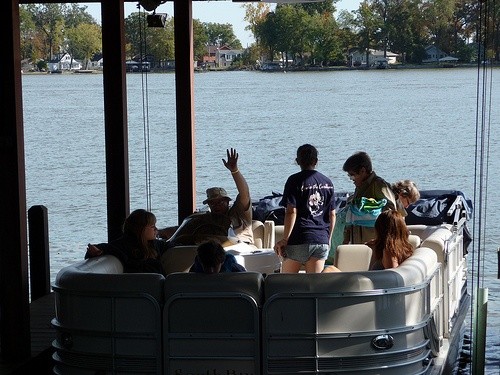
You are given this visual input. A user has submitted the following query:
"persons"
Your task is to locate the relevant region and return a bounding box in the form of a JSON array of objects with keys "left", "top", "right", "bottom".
[
  {"left": 187, "top": 148, "right": 258, "bottom": 251},
  {"left": 364, "top": 209, "right": 414, "bottom": 271},
  {"left": 274, "top": 144, "right": 337, "bottom": 274},
  {"left": 392, "top": 179, "right": 420, "bottom": 209},
  {"left": 85, "top": 209, "right": 184, "bottom": 277},
  {"left": 189, "top": 238, "right": 246, "bottom": 274},
  {"left": 342, "top": 152, "right": 397, "bottom": 244}
]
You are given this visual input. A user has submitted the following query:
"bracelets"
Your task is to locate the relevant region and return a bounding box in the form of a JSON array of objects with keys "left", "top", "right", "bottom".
[{"left": 231, "top": 169, "right": 239, "bottom": 173}]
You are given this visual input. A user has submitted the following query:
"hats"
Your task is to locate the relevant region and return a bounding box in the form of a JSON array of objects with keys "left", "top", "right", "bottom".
[{"left": 203, "top": 187, "right": 232, "bottom": 204}]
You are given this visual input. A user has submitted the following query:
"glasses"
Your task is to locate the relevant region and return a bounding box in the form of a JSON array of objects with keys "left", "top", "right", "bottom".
[{"left": 348, "top": 173, "right": 357, "bottom": 178}]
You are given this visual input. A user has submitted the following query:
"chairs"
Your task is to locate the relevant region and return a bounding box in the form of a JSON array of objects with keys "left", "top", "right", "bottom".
[{"left": 54, "top": 220, "right": 461, "bottom": 375}]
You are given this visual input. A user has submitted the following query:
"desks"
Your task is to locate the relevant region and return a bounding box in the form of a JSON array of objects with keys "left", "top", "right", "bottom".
[{"left": 224, "top": 243, "right": 281, "bottom": 272}]
